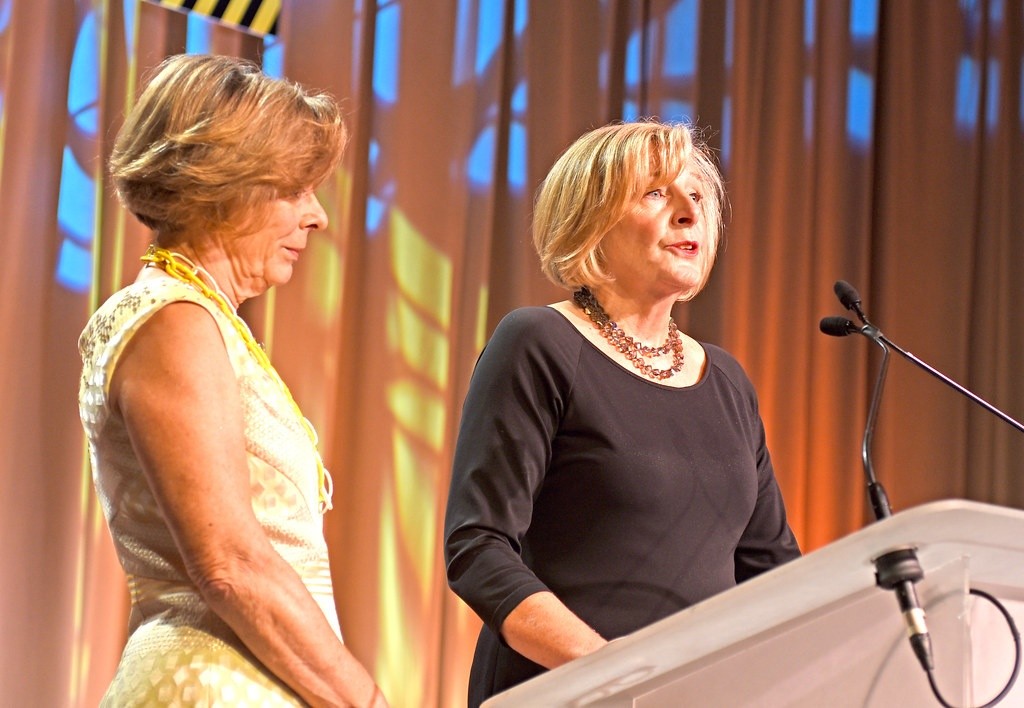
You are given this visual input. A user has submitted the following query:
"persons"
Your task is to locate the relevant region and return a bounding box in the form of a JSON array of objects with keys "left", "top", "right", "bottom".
[
  {"left": 444, "top": 125, "right": 803, "bottom": 708},
  {"left": 76, "top": 54, "right": 390, "bottom": 708}
]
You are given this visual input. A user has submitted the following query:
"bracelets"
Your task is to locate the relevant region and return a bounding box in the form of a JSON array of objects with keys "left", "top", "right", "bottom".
[{"left": 368, "top": 684, "right": 379, "bottom": 708}]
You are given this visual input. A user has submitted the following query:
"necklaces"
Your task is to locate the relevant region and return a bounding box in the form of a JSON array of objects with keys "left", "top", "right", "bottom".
[
  {"left": 140, "top": 244, "right": 333, "bottom": 514},
  {"left": 573, "top": 287, "right": 685, "bottom": 380}
]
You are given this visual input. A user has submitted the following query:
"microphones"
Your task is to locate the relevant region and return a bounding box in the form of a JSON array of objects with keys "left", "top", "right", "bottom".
[
  {"left": 834, "top": 280, "right": 1024, "bottom": 433},
  {"left": 819, "top": 316, "right": 891, "bottom": 522}
]
